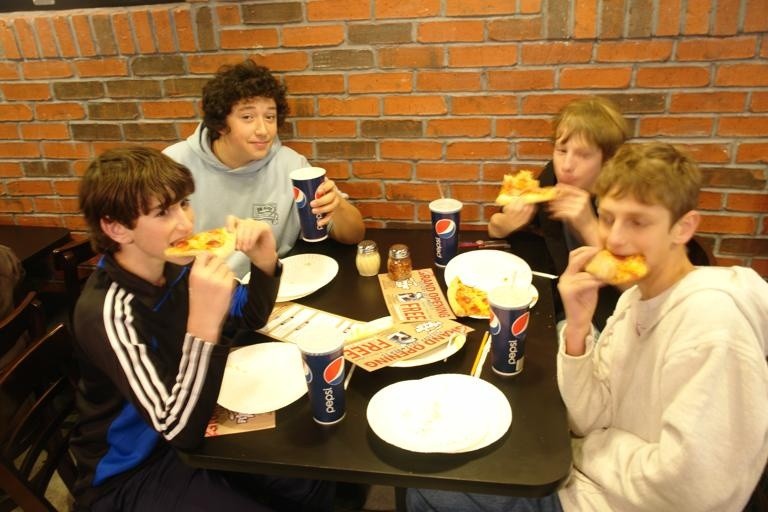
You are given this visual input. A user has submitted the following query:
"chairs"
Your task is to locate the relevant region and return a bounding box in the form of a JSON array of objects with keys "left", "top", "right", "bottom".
[
  {"left": 0, "top": 290, "right": 50, "bottom": 368},
  {"left": 54, "top": 233, "right": 92, "bottom": 317},
  {"left": 0, "top": 320, "right": 68, "bottom": 512}
]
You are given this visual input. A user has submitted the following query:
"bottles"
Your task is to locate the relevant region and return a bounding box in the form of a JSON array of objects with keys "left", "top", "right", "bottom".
[
  {"left": 355, "top": 240, "right": 381, "bottom": 277},
  {"left": 386, "top": 244, "right": 413, "bottom": 282}
]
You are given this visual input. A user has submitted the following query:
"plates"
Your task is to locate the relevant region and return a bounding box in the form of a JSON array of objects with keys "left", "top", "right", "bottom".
[
  {"left": 367, "top": 372, "right": 514, "bottom": 452},
  {"left": 239, "top": 252, "right": 339, "bottom": 305},
  {"left": 353, "top": 315, "right": 467, "bottom": 368},
  {"left": 218, "top": 338, "right": 308, "bottom": 416},
  {"left": 442, "top": 248, "right": 535, "bottom": 294},
  {"left": 465, "top": 284, "right": 539, "bottom": 320}
]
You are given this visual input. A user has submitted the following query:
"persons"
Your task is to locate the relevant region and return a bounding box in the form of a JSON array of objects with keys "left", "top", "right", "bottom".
[
  {"left": 155, "top": 59, "right": 365, "bottom": 278},
  {"left": 405, "top": 139, "right": 765, "bottom": 512},
  {"left": 487, "top": 98, "right": 702, "bottom": 330},
  {"left": 67, "top": 141, "right": 326, "bottom": 512}
]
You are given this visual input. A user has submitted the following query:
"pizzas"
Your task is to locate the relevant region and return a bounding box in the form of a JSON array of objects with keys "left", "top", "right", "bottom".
[
  {"left": 163, "top": 224, "right": 237, "bottom": 256},
  {"left": 583, "top": 246, "right": 649, "bottom": 288},
  {"left": 447, "top": 275, "right": 491, "bottom": 318},
  {"left": 496, "top": 169, "right": 560, "bottom": 208}
]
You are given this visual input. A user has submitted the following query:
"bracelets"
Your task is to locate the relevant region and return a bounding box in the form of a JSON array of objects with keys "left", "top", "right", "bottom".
[{"left": 255, "top": 251, "right": 277, "bottom": 268}]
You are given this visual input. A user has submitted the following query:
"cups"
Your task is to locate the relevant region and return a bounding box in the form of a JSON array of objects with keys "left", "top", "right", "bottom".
[
  {"left": 482, "top": 279, "right": 537, "bottom": 375},
  {"left": 290, "top": 167, "right": 332, "bottom": 242},
  {"left": 429, "top": 198, "right": 463, "bottom": 268},
  {"left": 297, "top": 325, "right": 348, "bottom": 426}
]
